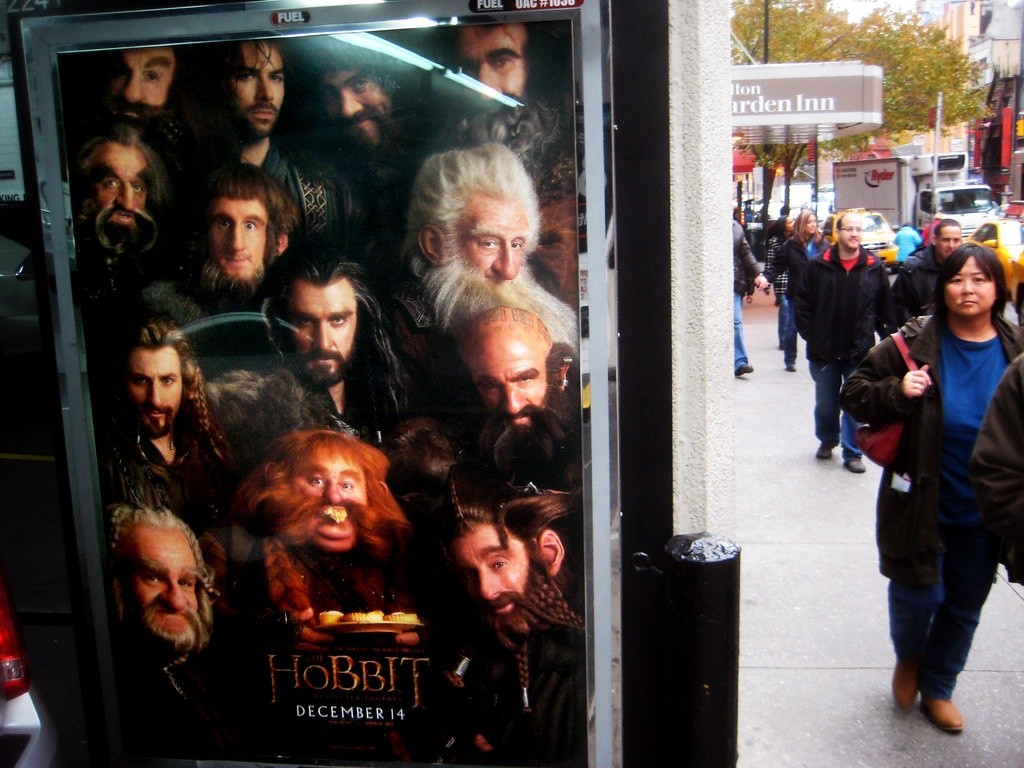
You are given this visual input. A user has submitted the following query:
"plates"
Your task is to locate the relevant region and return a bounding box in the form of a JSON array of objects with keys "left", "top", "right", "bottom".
[{"left": 310, "top": 620, "right": 426, "bottom": 633}]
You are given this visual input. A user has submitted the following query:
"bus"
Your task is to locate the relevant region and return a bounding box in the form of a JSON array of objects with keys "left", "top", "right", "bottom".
[{"left": 979, "top": 61, "right": 1024, "bottom": 204}]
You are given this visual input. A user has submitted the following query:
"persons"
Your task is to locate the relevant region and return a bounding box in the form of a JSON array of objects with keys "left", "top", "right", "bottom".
[
  {"left": 732, "top": 206, "right": 769, "bottom": 377},
  {"left": 70, "top": 25, "right": 588, "bottom": 768},
  {"left": 766, "top": 206, "right": 790, "bottom": 248},
  {"left": 759, "top": 210, "right": 830, "bottom": 372},
  {"left": 970, "top": 352, "right": 1024, "bottom": 587},
  {"left": 839, "top": 244, "right": 1024, "bottom": 733},
  {"left": 792, "top": 213, "right": 885, "bottom": 473},
  {"left": 889, "top": 219, "right": 963, "bottom": 329},
  {"left": 764, "top": 216, "right": 794, "bottom": 350}
]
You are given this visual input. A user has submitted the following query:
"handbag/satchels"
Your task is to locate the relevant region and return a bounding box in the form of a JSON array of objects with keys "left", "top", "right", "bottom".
[{"left": 853, "top": 331, "right": 917, "bottom": 467}]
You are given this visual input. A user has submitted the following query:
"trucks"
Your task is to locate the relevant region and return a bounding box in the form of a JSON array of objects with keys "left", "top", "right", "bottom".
[
  {"left": 779, "top": 183, "right": 812, "bottom": 208},
  {"left": 833, "top": 155, "right": 995, "bottom": 239}
]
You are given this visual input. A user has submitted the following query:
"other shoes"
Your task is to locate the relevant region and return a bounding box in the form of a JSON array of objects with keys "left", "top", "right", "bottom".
[
  {"left": 786, "top": 364, "right": 795, "bottom": 373},
  {"left": 816, "top": 439, "right": 836, "bottom": 460},
  {"left": 736, "top": 365, "right": 754, "bottom": 376},
  {"left": 844, "top": 457, "right": 866, "bottom": 474},
  {"left": 891, "top": 651, "right": 920, "bottom": 709},
  {"left": 921, "top": 690, "right": 963, "bottom": 731}
]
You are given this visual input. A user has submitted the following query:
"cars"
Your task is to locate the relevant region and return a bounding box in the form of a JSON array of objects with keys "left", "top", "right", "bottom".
[
  {"left": 176, "top": 311, "right": 283, "bottom": 380},
  {"left": 966, "top": 217, "right": 1024, "bottom": 327},
  {"left": 818, "top": 185, "right": 833, "bottom": 204},
  {"left": 822, "top": 212, "right": 901, "bottom": 274}
]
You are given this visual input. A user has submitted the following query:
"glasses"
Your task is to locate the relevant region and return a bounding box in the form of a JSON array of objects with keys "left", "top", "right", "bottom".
[{"left": 837, "top": 227, "right": 863, "bottom": 233}]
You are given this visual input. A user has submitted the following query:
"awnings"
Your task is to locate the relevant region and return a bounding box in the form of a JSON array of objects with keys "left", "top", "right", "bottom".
[{"left": 732, "top": 148, "right": 756, "bottom": 174}]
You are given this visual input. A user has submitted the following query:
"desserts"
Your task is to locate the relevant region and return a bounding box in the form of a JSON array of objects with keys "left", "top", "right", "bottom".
[
  {"left": 318, "top": 609, "right": 419, "bottom": 623},
  {"left": 323, "top": 506, "right": 347, "bottom": 523}
]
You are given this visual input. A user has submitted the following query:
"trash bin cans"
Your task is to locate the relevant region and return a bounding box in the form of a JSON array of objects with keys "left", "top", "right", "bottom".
[{"left": 744, "top": 227, "right": 766, "bottom": 262}]
